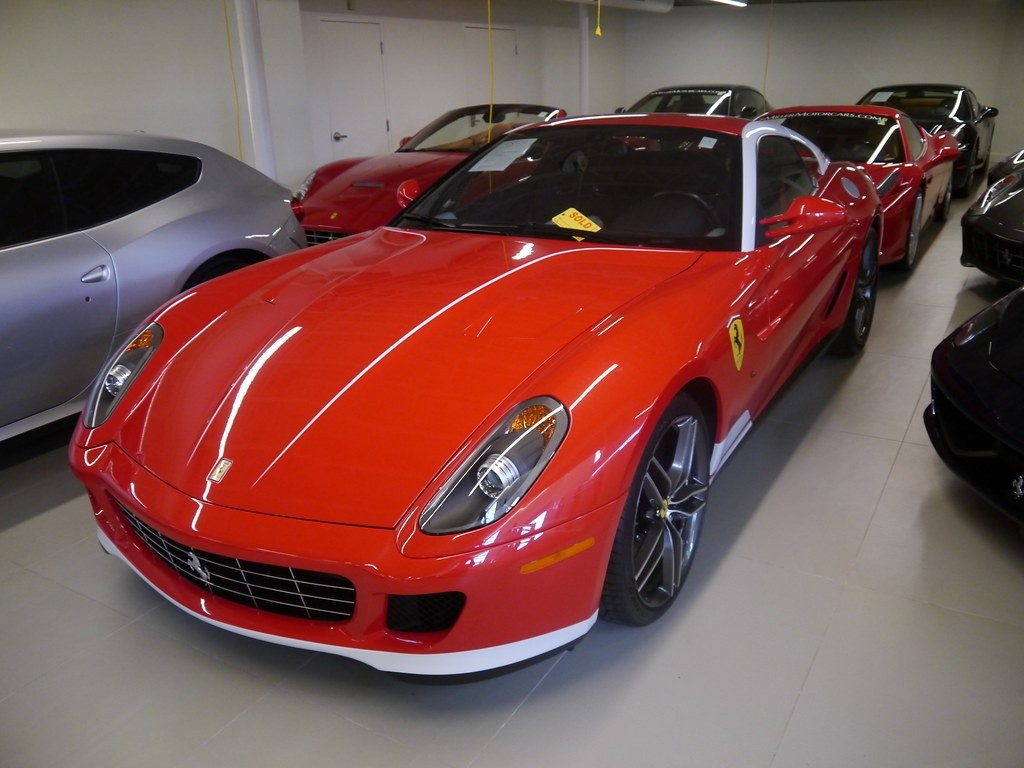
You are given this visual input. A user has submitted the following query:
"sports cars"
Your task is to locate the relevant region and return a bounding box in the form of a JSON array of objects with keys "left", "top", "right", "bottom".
[
  {"left": 747, "top": 104, "right": 961, "bottom": 273},
  {"left": 67, "top": 118, "right": 879, "bottom": 689},
  {"left": 297, "top": 103, "right": 570, "bottom": 252}
]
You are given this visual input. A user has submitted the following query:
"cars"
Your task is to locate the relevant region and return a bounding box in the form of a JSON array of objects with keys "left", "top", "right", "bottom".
[
  {"left": 1, "top": 130, "right": 306, "bottom": 447},
  {"left": 915, "top": 149, "right": 1024, "bottom": 529},
  {"left": 611, "top": 85, "right": 773, "bottom": 152},
  {"left": 856, "top": 83, "right": 1000, "bottom": 202}
]
